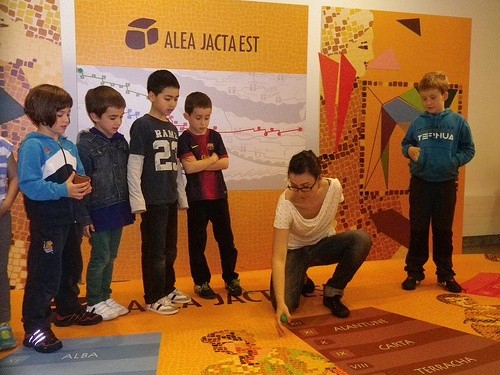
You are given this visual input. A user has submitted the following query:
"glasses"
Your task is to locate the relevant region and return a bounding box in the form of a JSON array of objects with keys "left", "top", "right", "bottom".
[{"left": 287, "top": 176, "right": 320, "bottom": 192}]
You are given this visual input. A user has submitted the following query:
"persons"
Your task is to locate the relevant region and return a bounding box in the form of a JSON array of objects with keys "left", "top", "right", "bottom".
[
  {"left": 0, "top": 135, "right": 19, "bottom": 351},
  {"left": 270, "top": 150, "right": 371, "bottom": 338},
  {"left": 127, "top": 70, "right": 190, "bottom": 314},
  {"left": 75, "top": 86, "right": 128, "bottom": 320},
  {"left": 177, "top": 92, "right": 243, "bottom": 298},
  {"left": 401, "top": 71, "right": 475, "bottom": 294},
  {"left": 17, "top": 84, "right": 103, "bottom": 352}
]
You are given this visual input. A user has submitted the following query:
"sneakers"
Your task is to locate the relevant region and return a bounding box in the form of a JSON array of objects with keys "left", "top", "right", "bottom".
[
  {"left": 193, "top": 282, "right": 216, "bottom": 300},
  {"left": 436, "top": 275, "right": 462, "bottom": 293},
  {"left": 304, "top": 273, "right": 315, "bottom": 294},
  {"left": 86, "top": 301, "right": 119, "bottom": 320},
  {"left": 168, "top": 289, "right": 191, "bottom": 304},
  {"left": 322, "top": 294, "right": 351, "bottom": 318},
  {"left": 104, "top": 298, "right": 129, "bottom": 316},
  {"left": 225, "top": 279, "right": 242, "bottom": 296},
  {"left": 401, "top": 273, "right": 425, "bottom": 291},
  {"left": 0, "top": 322, "right": 18, "bottom": 351},
  {"left": 22, "top": 325, "right": 63, "bottom": 353},
  {"left": 53, "top": 305, "right": 103, "bottom": 327},
  {"left": 147, "top": 295, "right": 181, "bottom": 315}
]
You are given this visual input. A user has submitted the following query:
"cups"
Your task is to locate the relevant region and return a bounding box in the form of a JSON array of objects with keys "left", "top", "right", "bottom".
[{"left": 73, "top": 174, "right": 90, "bottom": 186}]
